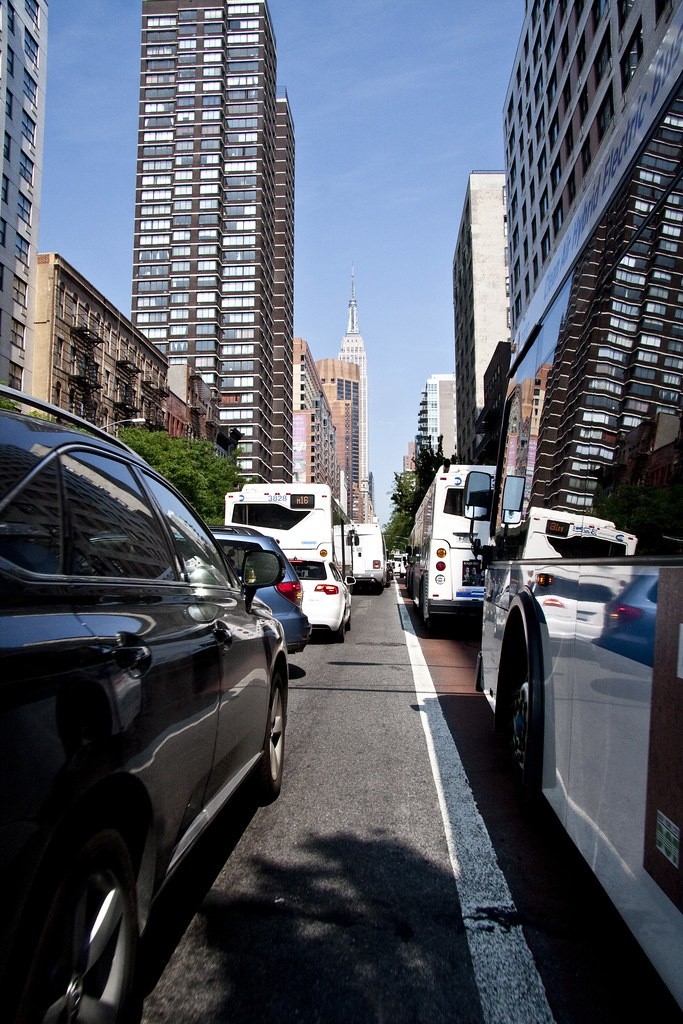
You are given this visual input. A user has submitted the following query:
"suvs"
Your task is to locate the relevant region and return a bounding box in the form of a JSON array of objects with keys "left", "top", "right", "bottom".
[
  {"left": 0, "top": 383, "right": 289, "bottom": 1023},
  {"left": 208, "top": 524, "right": 312, "bottom": 654}
]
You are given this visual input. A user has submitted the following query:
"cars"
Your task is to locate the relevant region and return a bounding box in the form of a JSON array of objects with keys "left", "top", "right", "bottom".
[
  {"left": 592, "top": 562, "right": 661, "bottom": 668},
  {"left": 288, "top": 555, "right": 356, "bottom": 642}
]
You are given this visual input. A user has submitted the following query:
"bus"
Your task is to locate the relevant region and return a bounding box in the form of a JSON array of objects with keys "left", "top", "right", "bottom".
[
  {"left": 498, "top": 506, "right": 638, "bottom": 639},
  {"left": 393, "top": 554, "right": 402, "bottom": 573},
  {"left": 410, "top": 464, "right": 497, "bottom": 638},
  {"left": 400, "top": 553, "right": 408, "bottom": 578},
  {"left": 224, "top": 484, "right": 359, "bottom": 592},
  {"left": 354, "top": 524, "right": 394, "bottom": 596},
  {"left": 473, "top": 0, "right": 683, "bottom": 1012}
]
